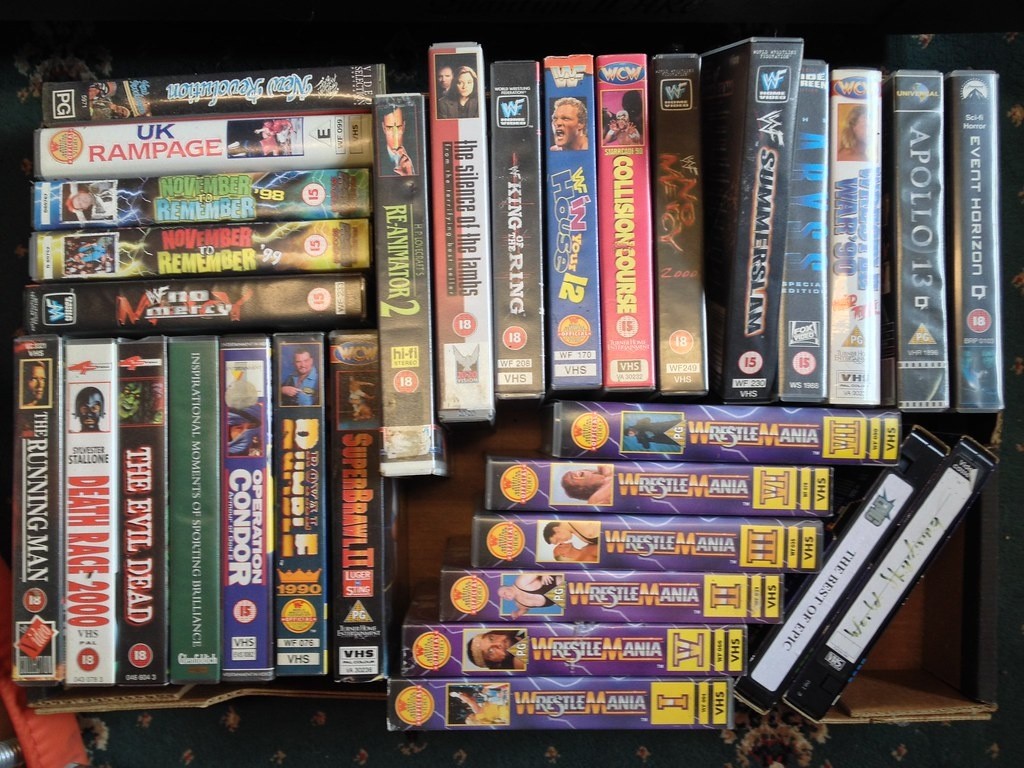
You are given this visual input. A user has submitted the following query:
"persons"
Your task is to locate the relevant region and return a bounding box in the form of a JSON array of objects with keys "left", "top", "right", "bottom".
[
  {"left": 281, "top": 348, "right": 319, "bottom": 405},
  {"left": 624, "top": 417, "right": 681, "bottom": 449},
  {"left": 21, "top": 361, "right": 46, "bottom": 407},
  {"left": 465, "top": 630, "right": 525, "bottom": 670},
  {"left": 227, "top": 403, "right": 261, "bottom": 456},
  {"left": 436, "top": 61, "right": 478, "bottom": 119},
  {"left": 549, "top": 97, "right": 588, "bottom": 150},
  {"left": 449, "top": 691, "right": 508, "bottom": 724},
  {"left": 62, "top": 236, "right": 114, "bottom": 275},
  {"left": 562, "top": 465, "right": 612, "bottom": 505},
  {"left": 543, "top": 521, "right": 599, "bottom": 562},
  {"left": 498, "top": 574, "right": 562, "bottom": 619},
  {"left": 68, "top": 182, "right": 113, "bottom": 220},
  {"left": 381, "top": 106, "right": 415, "bottom": 175}
]
